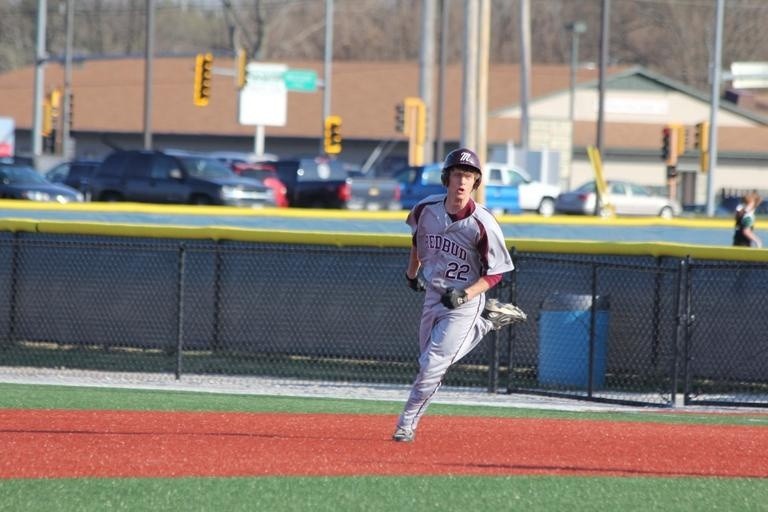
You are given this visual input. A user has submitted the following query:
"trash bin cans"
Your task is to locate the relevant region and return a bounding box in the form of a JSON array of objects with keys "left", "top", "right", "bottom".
[{"left": 537, "top": 293, "right": 610, "bottom": 391}]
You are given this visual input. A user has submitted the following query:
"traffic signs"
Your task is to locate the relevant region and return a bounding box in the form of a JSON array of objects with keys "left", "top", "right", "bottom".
[{"left": 282, "top": 70, "right": 316, "bottom": 92}]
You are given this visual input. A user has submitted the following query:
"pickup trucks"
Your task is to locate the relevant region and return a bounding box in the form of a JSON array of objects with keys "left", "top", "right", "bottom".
[{"left": 390, "top": 160, "right": 517, "bottom": 210}]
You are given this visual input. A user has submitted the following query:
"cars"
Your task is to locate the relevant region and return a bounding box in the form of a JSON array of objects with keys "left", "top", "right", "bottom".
[
  {"left": 556, "top": 176, "right": 683, "bottom": 221},
  {"left": 480, "top": 163, "right": 561, "bottom": 217}
]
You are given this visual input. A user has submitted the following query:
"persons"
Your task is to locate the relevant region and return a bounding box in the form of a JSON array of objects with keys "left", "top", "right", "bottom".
[
  {"left": 393, "top": 148, "right": 527, "bottom": 440},
  {"left": 731, "top": 188, "right": 762, "bottom": 249}
]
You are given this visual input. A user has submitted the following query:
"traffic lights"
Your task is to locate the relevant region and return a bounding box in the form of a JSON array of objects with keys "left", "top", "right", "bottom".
[
  {"left": 195, "top": 53, "right": 214, "bottom": 107},
  {"left": 661, "top": 128, "right": 671, "bottom": 161},
  {"left": 234, "top": 48, "right": 249, "bottom": 90},
  {"left": 693, "top": 123, "right": 706, "bottom": 151},
  {"left": 326, "top": 117, "right": 344, "bottom": 155},
  {"left": 391, "top": 103, "right": 405, "bottom": 134}
]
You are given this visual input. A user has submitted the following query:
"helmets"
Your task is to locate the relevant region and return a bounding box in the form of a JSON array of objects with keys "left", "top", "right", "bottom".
[{"left": 443, "top": 148, "right": 481, "bottom": 172}]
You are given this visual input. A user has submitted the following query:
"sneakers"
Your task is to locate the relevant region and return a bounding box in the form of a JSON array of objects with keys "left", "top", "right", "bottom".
[
  {"left": 393, "top": 426, "right": 417, "bottom": 443},
  {"left": 480, "top": 300, "right": 528, "bottom": 329}
]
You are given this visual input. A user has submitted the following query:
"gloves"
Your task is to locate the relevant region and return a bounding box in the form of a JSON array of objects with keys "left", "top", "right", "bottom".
[
  {"left": 403, "top": 272, "right": 424, "bottom": 291},
  {"left": 441, "top": 287, "right": 468, "bottom": 309}
]
